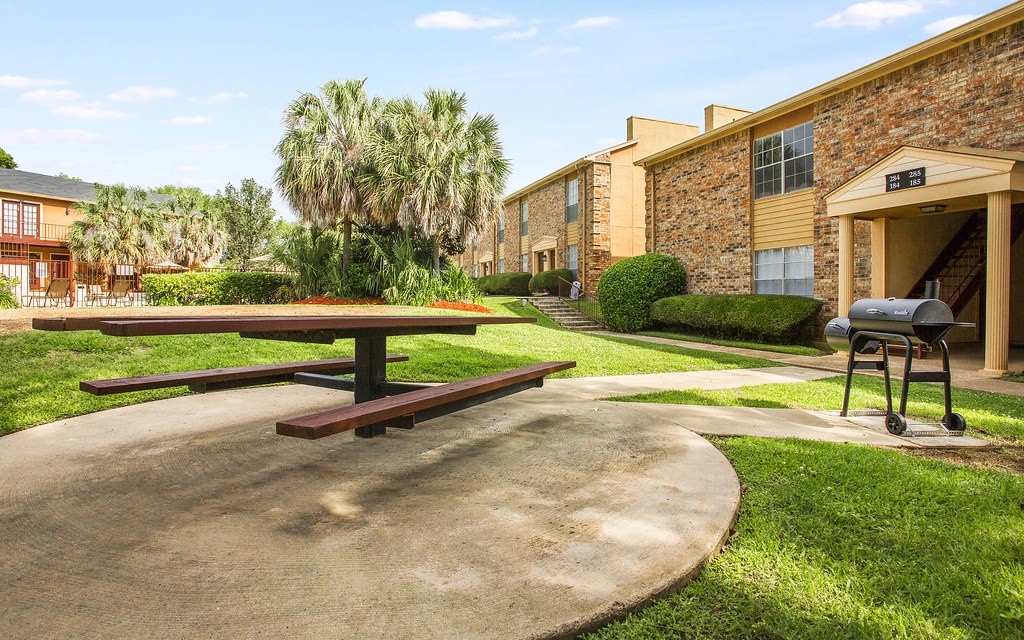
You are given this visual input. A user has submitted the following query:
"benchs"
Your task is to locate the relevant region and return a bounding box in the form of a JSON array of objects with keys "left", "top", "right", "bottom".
[{"left": 81, "top": 354, "right": 577, "bottom": 441}]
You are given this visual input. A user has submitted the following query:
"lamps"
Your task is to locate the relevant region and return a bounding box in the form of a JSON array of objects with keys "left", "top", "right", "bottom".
[
  {"left": 485, "top": 266, "right": 488, "bottom": 271},
  {"left": 541, "top": 255, "right": 547, "bottom": 265},
  {"left": 918, "top": 204, "right": 948, "bottom": 214}
]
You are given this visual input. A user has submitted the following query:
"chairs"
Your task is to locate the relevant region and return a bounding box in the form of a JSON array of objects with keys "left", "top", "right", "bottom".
[
  {"left": 83, "top": 280, "right": 136, "bottom": 307},
  {"left": 21, "top": 277, "right": 77, "bottom": 308}
]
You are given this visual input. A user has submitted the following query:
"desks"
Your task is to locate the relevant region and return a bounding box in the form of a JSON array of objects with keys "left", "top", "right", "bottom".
[{"left": 32, "top": 316, "right": 537, "bottom": 437}]
